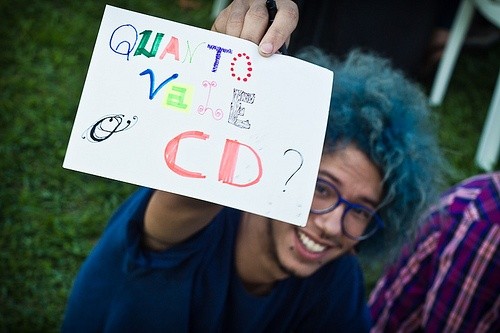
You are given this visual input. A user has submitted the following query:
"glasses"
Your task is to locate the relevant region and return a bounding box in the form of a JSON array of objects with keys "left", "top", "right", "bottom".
[{"left": 310, "top": 177, "right": 386, "bottom": 240}]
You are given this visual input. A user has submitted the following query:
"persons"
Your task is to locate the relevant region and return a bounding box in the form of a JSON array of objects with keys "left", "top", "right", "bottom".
[
  {"left": 61, "top": 0, "right": 442, "bottom": 333},
  {"left": 367, "top": 141, "right": 500, "bottom": 333}
]
